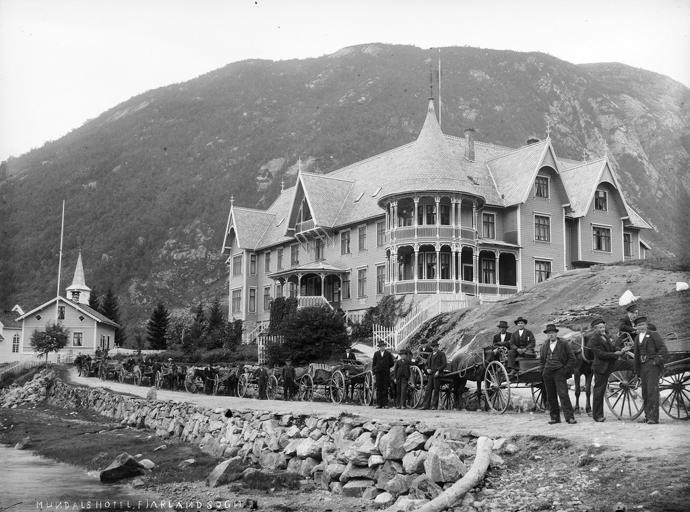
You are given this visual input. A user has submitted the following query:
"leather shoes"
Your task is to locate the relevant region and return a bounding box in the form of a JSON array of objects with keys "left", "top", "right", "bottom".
[
  {"left": 637, "top": 417, "right": 647, "bottom": 422},
  {"left": 376, "top": 402, "right": 436, "bottom": 410},
  {"left": 647, "top": 420, "right": 656, "bottom": 424},
  {"left": 548, "top": 420, "right": 561, "bottom": 424},
  {"left": 595, "top": 416, "right": 605, "bottom": 422},
  {"left": 567, "top": 418, "right": 577, "bottom": 424}
]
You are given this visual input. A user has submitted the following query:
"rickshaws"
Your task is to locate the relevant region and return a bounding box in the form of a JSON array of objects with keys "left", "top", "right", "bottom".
[{"left": 600, "top": 331, "right": 690, "bottom": 421}]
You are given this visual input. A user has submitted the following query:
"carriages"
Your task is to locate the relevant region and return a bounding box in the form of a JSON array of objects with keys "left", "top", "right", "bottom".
[
  {"left": 482, "top": 323, "right": 613, "bottom": 416},
  {"left": 406, "top": 339, "right": 497, "bottom": 410},
  {"left": 77, "top": 347, "right": 414, "bottom": 407}
]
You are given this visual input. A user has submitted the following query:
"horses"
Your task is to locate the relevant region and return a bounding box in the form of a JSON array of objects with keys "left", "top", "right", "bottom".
[
  {"left": 451, "top": 341, "right": 510, "bottom": 412},
  {"left": 541, "top": 323, "right": 616, "bottom": 418}
]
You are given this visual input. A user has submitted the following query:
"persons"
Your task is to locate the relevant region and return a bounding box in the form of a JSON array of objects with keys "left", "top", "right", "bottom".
[
  {"left": 539, "top": 324, "right": 577, "bottom": 425},
  {"left": 76, "top": 346, "right": 296, "bottom": 401},
  {"left": 619, "top": 305, "right": 656, "bottom": 341},
  {"left": 589, "top": 318, "right": 636, "bottom": 422},
  {"left": 493, "top": 321, "right": 512, "bottom": 350},
  {"left": 633, "top": 317, "right": 669, "bottom": 424},
  {"left": 506, "top": 317, "right": 535, "bottom": 376},
  {"left": 343, "top": 339, "right": 447, "bottom": 410}
]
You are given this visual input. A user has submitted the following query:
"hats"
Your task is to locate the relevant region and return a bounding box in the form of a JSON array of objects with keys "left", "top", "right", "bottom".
[
  {"left": 544, "top": 324, "right": 559, "bottom": 334},
  {"left": 497, "top": 321, "right": 510, "bottom": 328},
  {"left": 377, "top": 341, "right": 387, "bottom": 347},
  {"left": 514, "top": 317, "right": 528, "bottom": 325},
  {"left": 430, "top": 340, "right": 440, "bottom": 346},
  {"left": 398, "top": 349, "right": 408, "bottom": 355},
  {"left": 591, "top": 318, "right": 606, "bottom": 327},
  {"left": 634, "top": 316, "right": 649, "bottom": 325},
  {"left": 420, "top": 339, "right": 428, "bottom": 344},
  {"left": 626, "top": 304, "right": 639, "bottom": 313}
]
